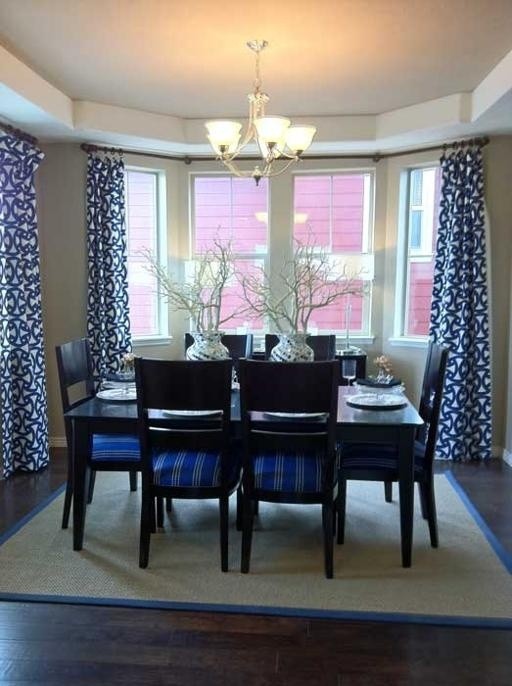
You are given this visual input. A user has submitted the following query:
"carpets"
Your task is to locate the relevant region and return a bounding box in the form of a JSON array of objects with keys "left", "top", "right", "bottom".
[{"left": 1, "top": 467, "right": 512, "bottom": 632}]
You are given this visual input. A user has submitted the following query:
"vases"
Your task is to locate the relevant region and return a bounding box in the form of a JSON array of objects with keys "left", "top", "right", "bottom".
[
  {"left": 184, "top": 334, "right": 231, "bottom": 360},
  {"left": 268, "top": 334, "right": 315, "bottom": 362}
]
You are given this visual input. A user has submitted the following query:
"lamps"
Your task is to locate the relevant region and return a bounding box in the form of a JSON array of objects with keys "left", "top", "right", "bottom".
[{"left": 204, "top": 39, "right": 315, "bottom": 187}]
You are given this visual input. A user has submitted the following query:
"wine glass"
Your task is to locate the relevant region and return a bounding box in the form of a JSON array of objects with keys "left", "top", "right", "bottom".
[{"left": 342, "top": 359, "right": 357, "bottom": 397}]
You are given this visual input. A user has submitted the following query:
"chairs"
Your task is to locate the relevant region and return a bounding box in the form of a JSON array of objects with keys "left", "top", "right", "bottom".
[
  {"left": 264, "top": 335, "right": 336, "bottom": 365},
  {"left": 337, "top": 341, "right": 448, "bottom": 553},
  {"left": 184, "top": 335, "right": 248, "bottom": 364},
  {"left": 133, "top": 355, "right": 243, "bottom": 573},
  {"left": 231, "top": 358, "right": 345, "bottom": 581},
  {"left": 52, "top": 338, "right": 142, "bottom": 531}
]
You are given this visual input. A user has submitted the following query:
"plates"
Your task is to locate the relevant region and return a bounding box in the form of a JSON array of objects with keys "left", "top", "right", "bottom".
[
  {"left": 345, "top": 393, "right": 407, "bottom": 408},
  {"left": 95, "top": 388, "right": 137, "bottom": 401}
]
